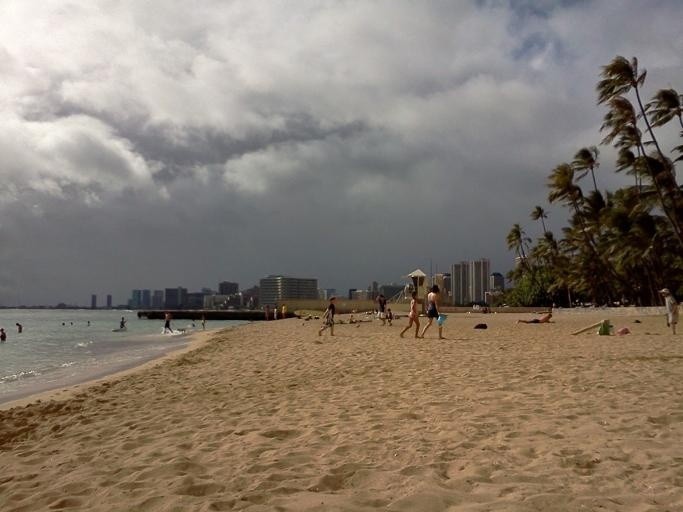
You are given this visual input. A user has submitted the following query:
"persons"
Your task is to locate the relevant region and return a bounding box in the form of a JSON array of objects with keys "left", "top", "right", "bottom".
[
  {"left": 420, "top": 285, "right": 447, "bottom": 339},
  {"left": 16, "top": 323, "right": 22, "bottom": 333},
  {"left": 281, "top": 303, "right": 287, "bottom": 318},
  {"left": 659, "top": 287, "right": 679, "bottom": 335},
  {"left": 318, "top": 296, "right": 337, "bottom": 336},
  {"left": 163, "top": 309, "right": 173, "bottom": 335},
  {"left": 120, "top": 315, "right": 128, "bottom": 328},
  {"left": 376, "top": 294, "right": 387, "bottom": 326},
  {"left": 399, "top": 291, "right": 420, "bottom": 338},
  {"left": 265, "top": 304, "right": 270, "bottom": 320},
  {"left": 517, "top": 313, "right": 555, "bottom": 324},
  {"left": 273, "top": 307, "right": 278, "bottom": 321},
  {"left": 0, "top": 328, "right": 7, "bottom": 340},
  {"left": 386, "top": 308, "right": 394, "bottom": 326}
]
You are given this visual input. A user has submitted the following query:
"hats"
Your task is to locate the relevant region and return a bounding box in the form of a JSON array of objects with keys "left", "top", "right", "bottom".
[
  {"left": 431, "top": 286, "right": 439, "bottom": 290},
  {"left": 659, "top": 288, "right": 669, "bottom": 293}
]
